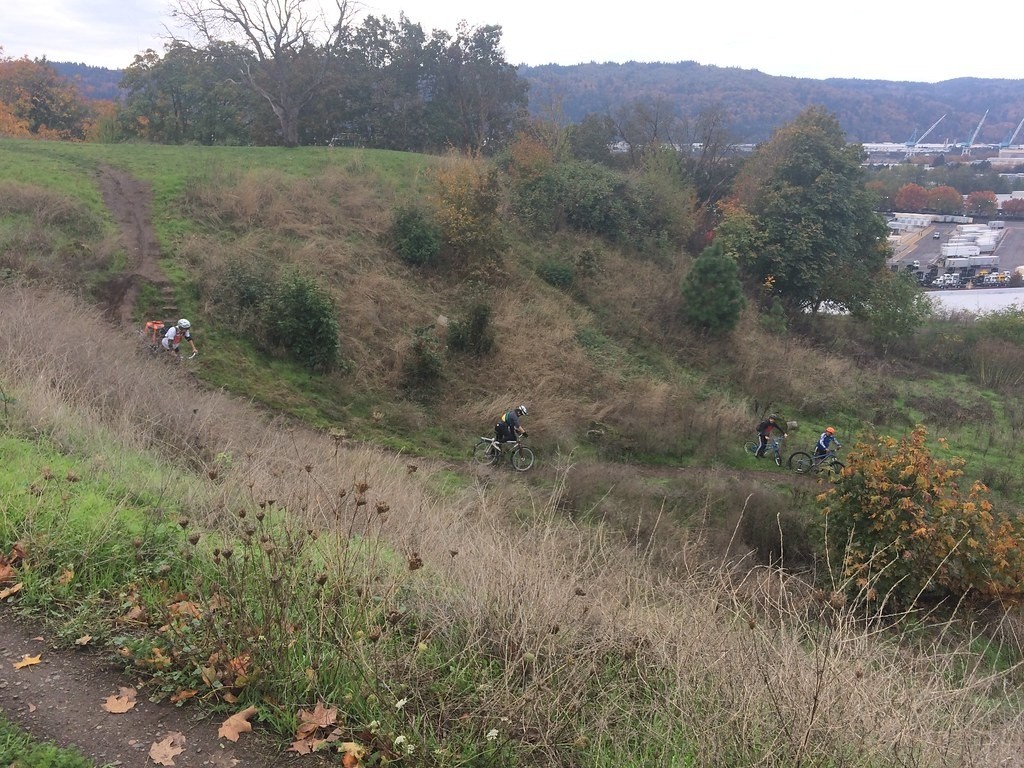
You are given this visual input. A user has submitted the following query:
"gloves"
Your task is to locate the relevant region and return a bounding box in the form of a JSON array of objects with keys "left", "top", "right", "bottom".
[{"left": 522, "top": 432, "right": 528, "bottom": 437}]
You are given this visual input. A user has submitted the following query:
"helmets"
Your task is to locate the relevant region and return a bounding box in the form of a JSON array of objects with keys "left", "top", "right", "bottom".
[
  {"left": 826, "top": 427, "right": 834, "bottom": 434},
  {"left": 769, "top": 414, "right": 776, "bottom": 420},
  {"left": 519, "top": 406, "right": 527, "bottom": 415},
  {"left": 178, "top": 319, "right": 190, "bottom": 329}
]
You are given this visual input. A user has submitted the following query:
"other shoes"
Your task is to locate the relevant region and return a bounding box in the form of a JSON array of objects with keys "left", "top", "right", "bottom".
[{"left": 491, "top": 442, "right": 502, "bottom": 452}]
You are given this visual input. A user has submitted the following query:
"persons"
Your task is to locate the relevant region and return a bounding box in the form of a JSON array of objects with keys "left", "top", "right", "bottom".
[
  {"left": 754, "top": 414, "right": 788, "bottom": 458},
  {"left": 491, "top": 405, "right": 528, "bottom": 459},
  {"left": 162, "top": 319, "right": 198, "bottom": 356},
  {"left": 813, "top": 427, "right": 842, "bottom": 475}
]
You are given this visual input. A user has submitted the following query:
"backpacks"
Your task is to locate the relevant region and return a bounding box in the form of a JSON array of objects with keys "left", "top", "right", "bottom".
[
  {"left": 756, "top": 421, "right": 768, "bottom": 431},
  {"left": 161, "top": 326, "right": 177, "bottom": 338}
]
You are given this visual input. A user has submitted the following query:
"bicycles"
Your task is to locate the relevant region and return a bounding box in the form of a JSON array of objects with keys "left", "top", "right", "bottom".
[
  {"left": 788, "top": 446, "right": 846, "bottom": 484},
  {"left": 140, "top": 343, "right": 200, "bottom": 394},
  {"left": 743, "top": 433, "right": 787, "bottom": 467},
  {"left": 473, "top": 430, "right": 534, "bottom": 471}
]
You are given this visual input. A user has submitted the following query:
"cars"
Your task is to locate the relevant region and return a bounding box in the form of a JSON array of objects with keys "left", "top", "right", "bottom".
[
  {"left": 801, "top": 299, "right": 874, "bottom": 315},
  {"left": 987, "top": 281, "right": 1001, "bottom": 286},
  {"left": 974, "top": 282, "right": 984, "bottom": 286}
]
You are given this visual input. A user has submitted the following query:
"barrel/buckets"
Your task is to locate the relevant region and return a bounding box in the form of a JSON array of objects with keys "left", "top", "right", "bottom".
[{"left": 786, "top": 421, "right": 798, "bottom": 430}]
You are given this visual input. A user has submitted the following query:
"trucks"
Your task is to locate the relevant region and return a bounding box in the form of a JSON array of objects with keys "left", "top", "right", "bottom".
[
  {"left": 933, "top": 232, "right": 940, "bottom": 239},
  {"left": 913, "top": 260, "right": 920, "bottom": 269}
]
